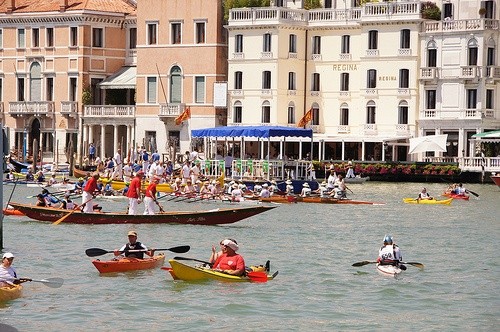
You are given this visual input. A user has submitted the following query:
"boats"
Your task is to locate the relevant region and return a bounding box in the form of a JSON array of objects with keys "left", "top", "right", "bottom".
[
  {"left": 376, "top": 259, "right": 403, "bottom": 278},
  {"left": 403, "top": 197, "right": 453, "bottom": 205},
  {"left": 168, "top": 259, "right": 278, "bottom": 281},
  {"left": 7, "top": 201, "right": 280, "bottom": 225},
  {"left": 443, "top": 192, "right": 470, "bottom": 200},
  {"left": 490, "top": 176, "right": 500, "bottom": 187},
  {"left": 0, "top": 285, "right": 24, "bottom": 302},
  {"left": 92, "top": 253, "right": 165, "bottom": 273},
  {"left": 3, "top": 155, "right": 382, "bottom": 205},
  {"left": 3, "top": 208, "right": 25, "bottom": 216}
]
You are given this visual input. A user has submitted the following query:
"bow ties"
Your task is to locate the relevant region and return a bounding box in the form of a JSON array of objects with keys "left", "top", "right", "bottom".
[{"left": 130, "top": 244, "right": 134, "bottom": 246}]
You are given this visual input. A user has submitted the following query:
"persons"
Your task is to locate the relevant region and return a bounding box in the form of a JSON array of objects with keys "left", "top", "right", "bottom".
[
  {"left": 379, "top": 236, "right": 401, "bottom": 266},
  {"left": 127, "top": 170, "right": 145, "bottom": 215},
  {"left": 4, "top": 158, "right": 62, "bottom": 185},
  {"left": 417, "top": 188, "right": 432, "bottom": 200},
  {"left": 80, "top": 142, "right": 363, "bottom": 203},
  {"left": 58, "top": 192, "right": 78, "bottom": 210},
  {"left": 207, "top": 239, "right": 225, "bottom": 271},
  {"left": 36, "top": 193, "right": 49, "bottom": 208},
  {"left": 143, "top": 177, "right": 160, "bottom": 215},
  {"left": 41, "top": 189, "right": 58, "bottom": 207},
  {"left": 454, "top": 183, "right": 469, "bottom": 196},
  {"left": 212, "top": 238, "right": 245, "bottom": 277},
  {"left": 0, "top": 252, "right": 27, "bottom": 289},
  {"left": 81, "top": 172, "right": 100, "bottom": 213},
  {"left": 113, "top": 231, "right": 156, "bottom": 260}
]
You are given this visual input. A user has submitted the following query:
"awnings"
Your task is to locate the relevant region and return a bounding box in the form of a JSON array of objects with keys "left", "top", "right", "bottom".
[{"left": 97, "top": 67, "right": 136, "bottom": 89}]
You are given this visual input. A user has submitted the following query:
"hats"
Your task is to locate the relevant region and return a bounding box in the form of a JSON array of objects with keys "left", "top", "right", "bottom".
[
  {"left": 3, "top": 252, "right": 15, "bottom": 259},
  {"left": 286, "top": 179, "right": 292, "bottom": 184},
  {"left": 176, "top": 177, "right": 277, "bottom": 190},
  {"left": 127, "top": 230, "right": 138, "bottom": 237},
  {"left": 384, "top": 234, "right": 393, "bottom": 242},
  {"left": 137, "top": 169, "right": 145, "bottom": 176},
  {"left": 302, "top": 182, "right": 309, "bottom": 187},
  {"left": 153, "top": 177, "right": 160, "bottom": 181}
]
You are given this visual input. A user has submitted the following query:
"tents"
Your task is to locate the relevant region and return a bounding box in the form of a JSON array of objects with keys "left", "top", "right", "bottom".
[
  {"left": 190, "top": 127, "right": 313, "bottom": 178},
  {"left": 468, "top": 139, "right": 500, "bottom": 166},
  {"left": 210, "top": 133, "right": 413, "bottom": 161}
]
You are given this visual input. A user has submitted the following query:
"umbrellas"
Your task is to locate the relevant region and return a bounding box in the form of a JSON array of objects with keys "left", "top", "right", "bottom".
[
  {"left": 408, "top": 134, "right": 448, "bottom": 154},
  {"left": 470, "top": 131, "right": 500, "bottom": 137}
]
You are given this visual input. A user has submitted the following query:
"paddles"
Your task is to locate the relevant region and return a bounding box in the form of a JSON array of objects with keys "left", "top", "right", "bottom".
[
  {"left": 26, "top": 190, "right": 74, "bottom": 198},
  {"left": 469, "top": 191, "right": 479, "bottom": 197},
  {"left": 345, "top": 185, "right": 353, "bottom": 194},
  {"left": 168, "top": 196, "right": 179, "bottom": 201},
  {"left": 0, "top": 277, "right": 64, "bottom": 288},
  {"left": 38, "top": 184, "right": 45, "bottom": 189},
  {"left": 188, "top": 198, "right": 209, "bottom": 204},
  {"left": 225, "top": 271, "right": 268, "bottom": 283},
  {"left": 174, "top": 256, "right": 212, "bottom": 265},
  {"left": 155, "top": 199, "right": 165, "bottom": 212},
  {"left": 175, "top": 198, "right": 190, "bottom": 202},
  {"left": 85, "top": 246, "right": 190, "bottom": 257},
  {"left": 157, "top": 190, "right": 179, "bottom": 199},
  {"left": 351, "top": 260, "right": 424, "bottom": 269},
  {"left": 53, "top": 191, "right": 100, "bottom": 224}
]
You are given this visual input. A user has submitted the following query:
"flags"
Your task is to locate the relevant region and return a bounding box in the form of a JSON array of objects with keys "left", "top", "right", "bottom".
[
  {"left": 174, "top": 105, "right": 191, "bottom": 125},
  {"left": 297, "top": 106, "right": 313, "bottom": 128}
]
